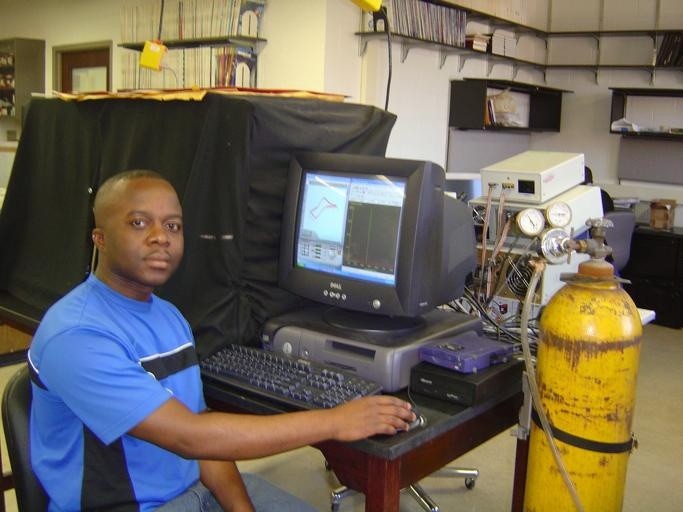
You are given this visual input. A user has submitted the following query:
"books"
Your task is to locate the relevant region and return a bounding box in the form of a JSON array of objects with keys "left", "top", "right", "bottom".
[
  {"left": 485, "top": 98, "right": 496, "bottom": 126},
  {"left": 656, "top": 33, "right": 683, "bottom": 68},
  {"left": 364, "top": 1, "right": 517, "bottom": 59},
  {"left": 111, "top": 1, "right": 264, "bottom": 90}
]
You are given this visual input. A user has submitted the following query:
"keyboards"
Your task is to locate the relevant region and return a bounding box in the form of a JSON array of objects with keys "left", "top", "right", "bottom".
[{"left": 197, "top": 340, "right": 384, "bottom": 410}]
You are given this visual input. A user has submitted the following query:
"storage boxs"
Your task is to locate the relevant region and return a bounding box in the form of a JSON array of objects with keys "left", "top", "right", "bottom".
[{"left": 651, "top": 200, "right": 677, "bottom": 230}]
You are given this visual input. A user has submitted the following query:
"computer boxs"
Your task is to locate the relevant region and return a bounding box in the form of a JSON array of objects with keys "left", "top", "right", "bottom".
[{"left": 259, "top": 306, "right": 482, "bottom": 393}]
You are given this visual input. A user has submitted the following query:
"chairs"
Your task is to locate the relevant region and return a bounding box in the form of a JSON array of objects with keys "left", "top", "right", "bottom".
[{"left": 3, "top": 364, "right": 50, "bottom": 512}]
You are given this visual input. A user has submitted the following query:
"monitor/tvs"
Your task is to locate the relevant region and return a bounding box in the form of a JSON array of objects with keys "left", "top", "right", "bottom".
[{"left": 276, "top": 151, "right": 480, "bottom": 334}]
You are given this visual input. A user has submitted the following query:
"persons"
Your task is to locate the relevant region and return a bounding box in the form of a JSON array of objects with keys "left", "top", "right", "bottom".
[
  {"left": 580, "top": 167, "right": 613, "bottom": 213},
  {"left": 26, "top": 171, "right": 417, "bottom": 512}
]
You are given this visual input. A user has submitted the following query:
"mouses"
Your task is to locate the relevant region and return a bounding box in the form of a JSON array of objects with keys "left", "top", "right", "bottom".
[{"left": 392, "top": 407, "right": 422, "bottom": 431}]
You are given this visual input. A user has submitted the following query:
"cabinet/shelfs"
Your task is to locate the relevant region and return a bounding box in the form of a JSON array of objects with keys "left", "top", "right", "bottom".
[
  {"left": 443, "top": 74, "right": 579, "bottom": 136},
  {"left": 606, "top": 85, "right": 683, "bottom": 140},
  {"left": 354, "top": 0, "right": 683, "bottom": 84},
  {"left": 117, "top": 35, "right": 267, "bottom": 95},
  {"left": 1, "top": 38, "right": 44, "bottom": 124},
  {"left": 620, "top": 222, "right": 683, "bottom": 331}
]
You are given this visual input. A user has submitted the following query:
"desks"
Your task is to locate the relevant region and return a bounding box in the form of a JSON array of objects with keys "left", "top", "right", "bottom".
[{"left": 1, "top": 268, "right": 530, "bottom": 512}]
